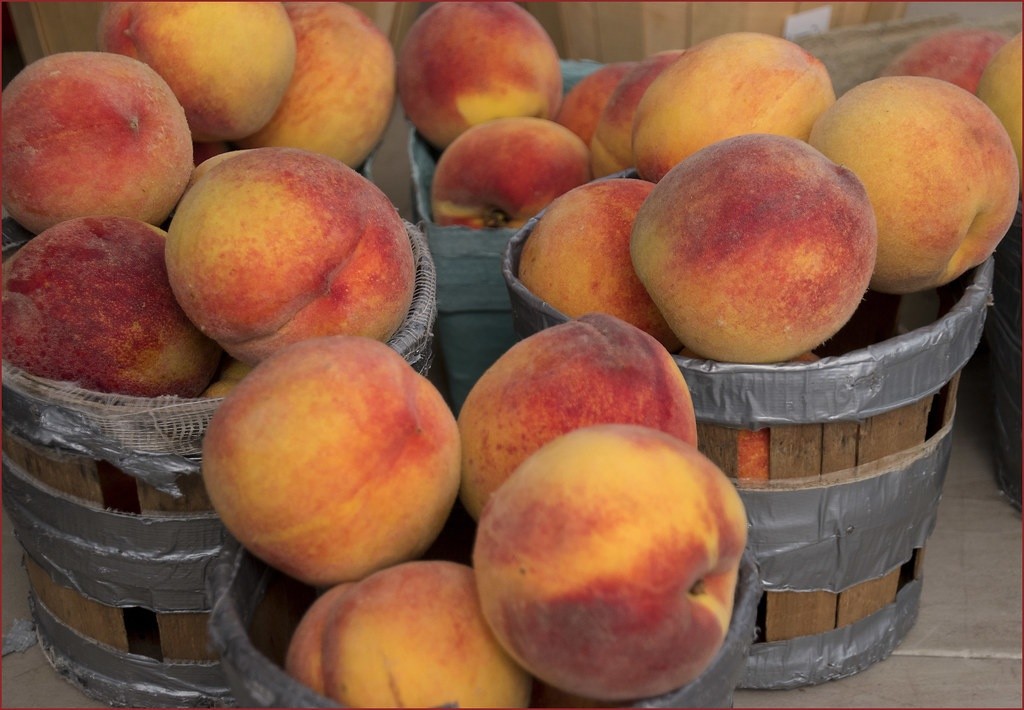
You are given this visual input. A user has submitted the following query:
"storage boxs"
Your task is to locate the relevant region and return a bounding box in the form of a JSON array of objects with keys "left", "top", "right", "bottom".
[
  {"left": 9, "top": 2, "right": 417, "bottom": 73},
  {"left": 530, "top": 2, "right": 907, "bottom": 63},
  {"left": 407, "top": 64, "right": 609, "bottom": 404}
]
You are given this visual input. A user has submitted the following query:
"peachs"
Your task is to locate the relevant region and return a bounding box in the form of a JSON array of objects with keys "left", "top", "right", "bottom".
[{"left": 2, "top": 2, "right": 1022, "bottom": 710}]
